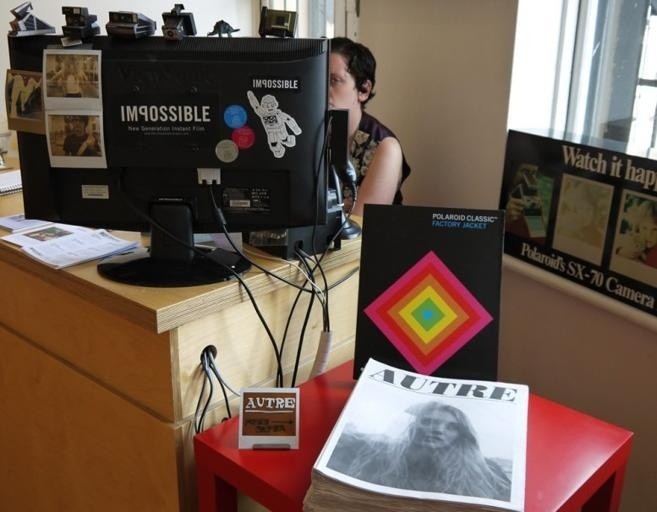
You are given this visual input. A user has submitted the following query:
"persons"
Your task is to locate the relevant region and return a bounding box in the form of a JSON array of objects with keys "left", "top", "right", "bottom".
[
  {"left": 63, "top": 115, "right": 98, "bottom": 156},
  {"left": 46, "top": 55, "right": 99, "bottom": 98},
  {"left": 327, "top": 37, "right": 411, "bottom": 218},
  {"left": 504, "top": 198, "right": 526, "bottom": 222},
  {"left": 92, "top": 132, "right": 101, "bottom": 144},
  {"left": 615, "top": 199, "right": 657, "bottom": 268}
]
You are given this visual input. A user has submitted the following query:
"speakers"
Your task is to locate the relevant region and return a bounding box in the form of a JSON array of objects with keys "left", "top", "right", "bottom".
[{"left": 242, "top": 109, "right": 349, "bottom": 262}]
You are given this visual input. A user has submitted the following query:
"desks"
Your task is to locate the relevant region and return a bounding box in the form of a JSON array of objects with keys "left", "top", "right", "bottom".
[
  {"left": 0, "top": 156, "right": 376, "bottom": 512},
  {"left": 193, "top": 355, "right": 637, "bottom": 512}
]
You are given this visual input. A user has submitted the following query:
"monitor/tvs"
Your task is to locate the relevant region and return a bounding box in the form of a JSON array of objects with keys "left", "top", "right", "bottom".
[{"left": 7, "top": 35, "right": 329, "bottom": 288}]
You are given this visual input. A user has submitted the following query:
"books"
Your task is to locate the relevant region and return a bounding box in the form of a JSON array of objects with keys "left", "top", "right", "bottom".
[
  {"left": 0, "top": 169, "right": 23, "bottom": 198},
  {"left": 302, "top": 356, "right": 531, "bottom": 511},
  {"left": 0, "top": 214, "right": 140, "bottom": 271}
]
